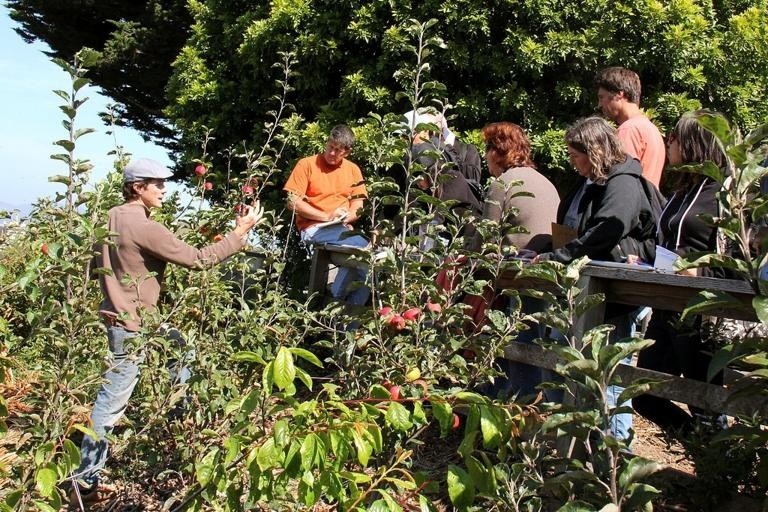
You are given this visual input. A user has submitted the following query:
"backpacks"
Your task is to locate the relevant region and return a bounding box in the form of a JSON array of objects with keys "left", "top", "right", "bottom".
[{"left": 632, "top": 176, "right": 670, "bottom": 258}]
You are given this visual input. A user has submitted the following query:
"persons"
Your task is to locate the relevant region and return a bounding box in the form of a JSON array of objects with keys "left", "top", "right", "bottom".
[
  {"left": 532, "top": 117, "right": 658, "bottom": 449},
  {"left": 390, "top": 111, "right": 483, "bottom": 318},
  {"left": 281, "top": 125, "right": 382, "bottom": 329},
  {"left": 659, "top": 116, "right": 733, "bottom": 444},
  {"left": 484, "top": 121, "right": 562, "bottom": 400},
  {"left": 597, "top": 67, "right": 666, "bottom": 185},
  {"left": 69, "top": 159, "right": 268, "bottom": 504}
]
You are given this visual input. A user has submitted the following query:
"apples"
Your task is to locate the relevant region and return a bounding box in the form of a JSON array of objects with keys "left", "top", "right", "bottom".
[{"left": 40, "top": 165, "right": 468, "bottom": 429}]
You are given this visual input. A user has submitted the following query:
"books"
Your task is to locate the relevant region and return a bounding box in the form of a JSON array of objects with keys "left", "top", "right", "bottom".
[{"left": 316, "top": 211, "right": 348, "bottom": 228}]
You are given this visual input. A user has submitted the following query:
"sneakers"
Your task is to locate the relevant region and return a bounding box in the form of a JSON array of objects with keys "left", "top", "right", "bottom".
[{"left": 70, "top": 483, "right": 114, "bottom": 508}]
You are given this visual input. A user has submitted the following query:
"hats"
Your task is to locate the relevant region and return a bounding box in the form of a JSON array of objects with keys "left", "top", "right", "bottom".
[{"left": 120, "top": 156, "right": 176, "bottom": 183}]
